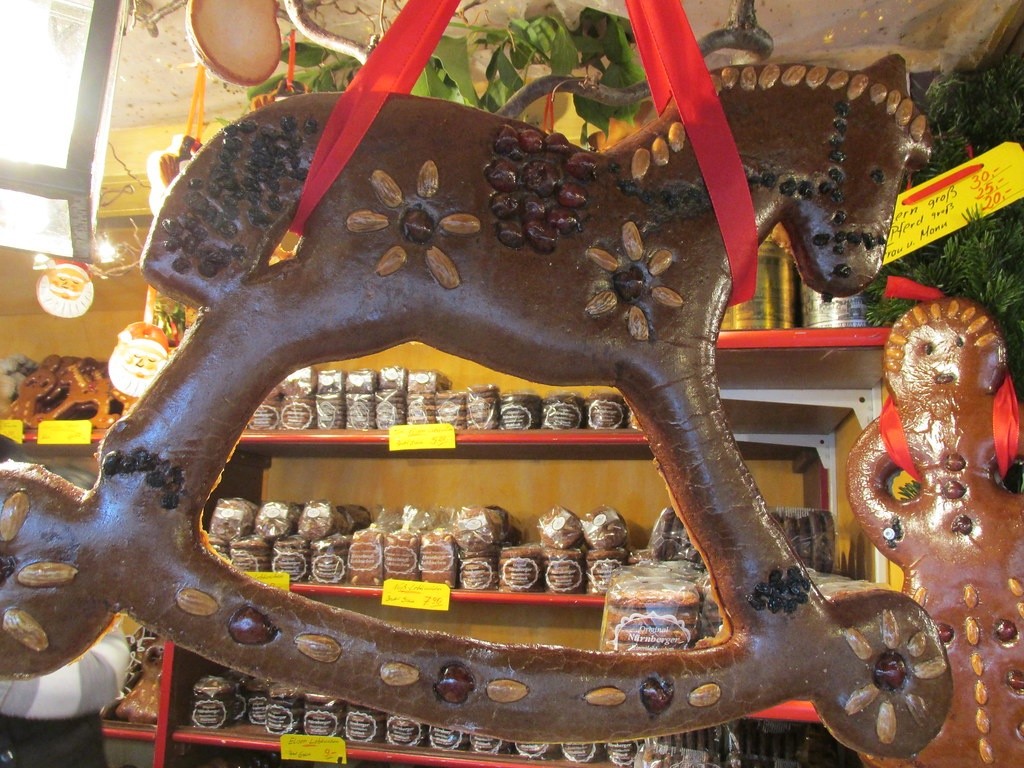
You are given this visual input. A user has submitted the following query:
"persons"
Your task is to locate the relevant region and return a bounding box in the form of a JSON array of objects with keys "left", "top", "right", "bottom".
[{"left": 0, "top": 435, "right": 132, "bottom": 768}]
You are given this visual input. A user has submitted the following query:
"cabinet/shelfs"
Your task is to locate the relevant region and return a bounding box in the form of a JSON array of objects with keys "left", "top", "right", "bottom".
[
  {"left": 101, "top": 427, "right": 700, "bottom": 767},
  {"left": 705, "top": 326, "right": 897, "bottom": 722}
]
